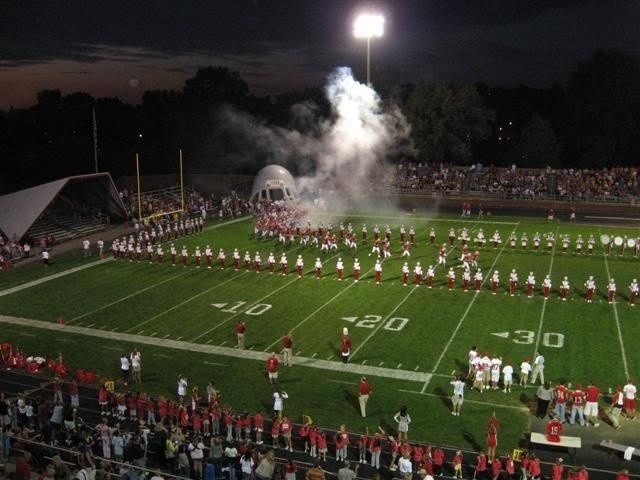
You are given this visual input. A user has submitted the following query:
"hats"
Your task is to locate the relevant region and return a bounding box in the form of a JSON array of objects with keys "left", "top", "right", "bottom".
[
  {"left": 623, "top": 469, "right": 629, "bottom": 475},
  {"left": 417, "top": 468, "right": 427, "bottom": 475}
]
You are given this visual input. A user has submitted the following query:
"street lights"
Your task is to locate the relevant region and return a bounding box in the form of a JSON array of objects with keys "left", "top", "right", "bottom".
[{"left": 350, "top": 12, "right": 384, "bottom": 86}]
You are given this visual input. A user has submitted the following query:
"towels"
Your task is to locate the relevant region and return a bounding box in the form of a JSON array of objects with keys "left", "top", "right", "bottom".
[{"left": 623, "top": 446, "right": 635, "bottom": 461}]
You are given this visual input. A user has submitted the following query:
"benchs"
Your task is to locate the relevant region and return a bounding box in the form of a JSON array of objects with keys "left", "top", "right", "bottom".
[
  {"left": 365, "top": 162, "right": 639, "bottom": 205},
  {"left": 0, "top": 184, "right": 217, "bottom": 267},
  {"left": 530, "top": 431, "right": 583, "bottom": 456},
  {"left": 600, "top": 439, "right": 640, "bottom": 461}
]
